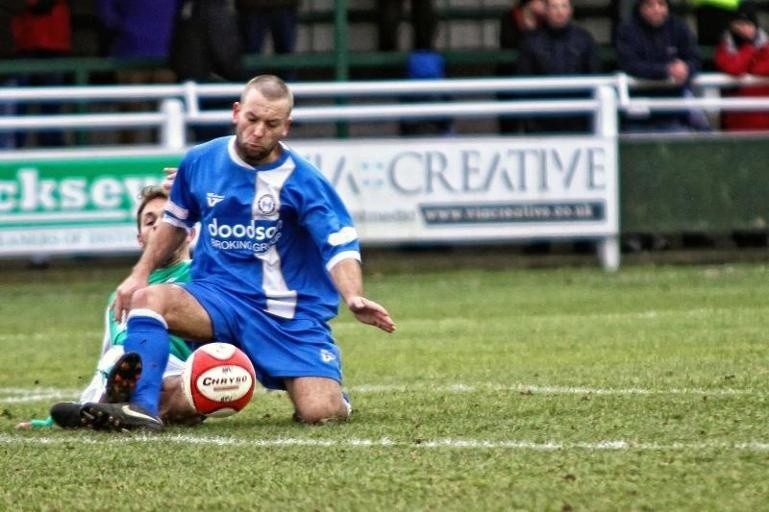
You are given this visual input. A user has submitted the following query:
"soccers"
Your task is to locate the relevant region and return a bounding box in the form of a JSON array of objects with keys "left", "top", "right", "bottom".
[{"left": 181, "top": 342, "right": 256, "bottom": 418}]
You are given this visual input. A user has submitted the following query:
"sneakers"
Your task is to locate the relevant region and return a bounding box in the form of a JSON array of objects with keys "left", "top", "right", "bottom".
[{"left": 52, "top": 353, "right": 164, "bottom": 432}]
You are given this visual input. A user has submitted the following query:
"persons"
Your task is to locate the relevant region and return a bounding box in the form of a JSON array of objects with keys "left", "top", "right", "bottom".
[
  {"left": 714, "top": 10, "right": 768, "bottom": 132},
  {"left": 495, "top": 1, "right": 601, "bottom": 133},
  {"left": 499, "top": 2, "right": 551, "bottom": 48},
  {"left": 81, "top": 74, "right": 396, "bottom": 432},
  {"left": 12, "top": 167, "right": 208, "bottom": 429},
  {"left": 375, "top": 2, "right": 437, "bottom": 52},
  {"left": 175, "top": 1, "right": 248, "bottom": 145},
  {"left": 86, "top": 2, "right": 176, "bottom": 146},
  {"left": 237, "top": 1, "right": 300, "bottom": 80},
  {"left": 9, "top": 0, "right": 73, "bottom": 148},
  {"left": 613, "top": 1, "right": 714, "bottom": 132}
]
